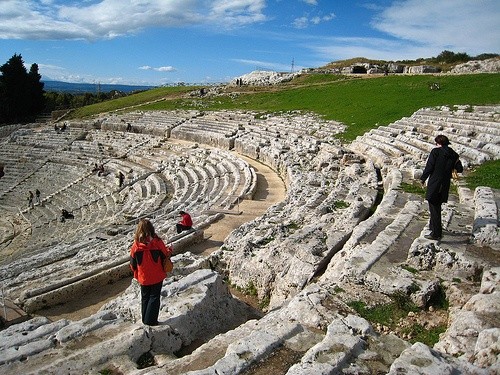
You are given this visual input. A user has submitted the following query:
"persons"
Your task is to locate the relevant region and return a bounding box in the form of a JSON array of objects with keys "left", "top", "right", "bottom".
[
  {"left": 126, "top": 123, "right": 131, "bottom": 132},
  {"left": 0, "top": 166, "right": 5, "bottom": 179},
  {"left": 36, "top": 189, "right": 41, "bottom": 202},
  {"left": 420, "top": 134, "right": 464, "bottom": 241},
  {"left": 54, "top": 123, "right": 68, "bottom": 132},
  {"left": 27, "top": 191, "right": 34, "bottom": 209},
  {"left": 92, "top": 163, "right": 124, "bottom": 187},
  {"left": 130, "top": 219, "right": 174, "bottom": 325},
  {"left": 61, "top": 209, "right": 68, "bottom": 222},
  {"left": 176, "top": 211, "right": 193, "bottom": 234}
]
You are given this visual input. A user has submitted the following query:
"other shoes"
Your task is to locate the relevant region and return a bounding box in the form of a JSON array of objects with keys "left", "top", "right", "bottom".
[{"left": 424, "top": 235, "right": 441, "bottom": 240}]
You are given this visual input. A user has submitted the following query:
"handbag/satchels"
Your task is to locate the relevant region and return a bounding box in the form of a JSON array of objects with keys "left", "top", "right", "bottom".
[
  {"left": 451, "top": 167, "right": 458, "bottom": 180},
  {"left": 162, "top": 252, "right": 174, "bottom": 273}
]
